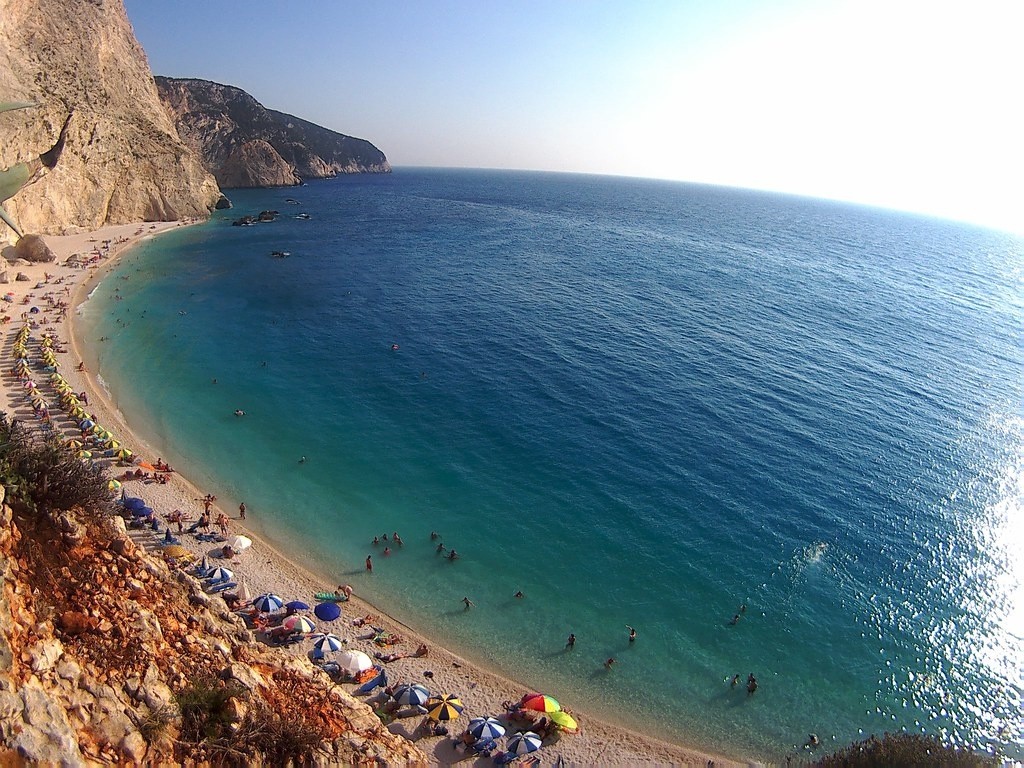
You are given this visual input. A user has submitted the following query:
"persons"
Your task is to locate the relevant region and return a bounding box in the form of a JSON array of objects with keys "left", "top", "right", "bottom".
[
  {"left": 810, "top": 733, "right": 820, "bottom": 747},
  {"left": 20, "top": 235, "right": 306, "bottom": 487},
  {"left": 626, "top": 624, "right": 636, "bottom": 642},
  {"left": 730, "top": 604, "right": 758, "bottom": 693},
  {"left": 565, "top": 634, "right": 575, "bottom": 650},
  {"left": 604, "top": 657, "right": 618, "bottom": 670},
  {"left": 163, "top": 494, "right": 554, "bottom": 757}
]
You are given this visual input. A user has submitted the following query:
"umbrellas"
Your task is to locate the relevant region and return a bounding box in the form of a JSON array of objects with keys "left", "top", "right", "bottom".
[
  {"left": 336, "top": 650, "right": 373, "bottom": 671},
  {"left": 467, "top": 717, "right": 505, "bottom": 740},
  {"left": 153, "top": 519, "right": 340, "bottom": 640},
  {"left": 392, "top": 683, "right": 431, "bottom": 706},
  {"left": 310, "top": 633, "right": 342, "bottom": 652},
  {"left": 378, "top": 669, "right": 387, "bottom": 688},
  {"left": 505, "top": 730, "right": 543, "bottom": 755},
  {"left": 520, "top": 693, "right": 580, "bottom": 731},
  {"left": 3, "top": 292, "right": 153, "bottom": 516},
  {"left": 427, "top": 692, "right": 463, "bottom": 720}
]
